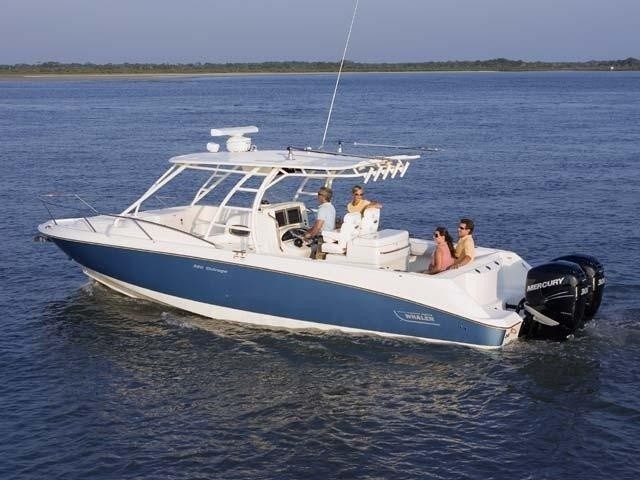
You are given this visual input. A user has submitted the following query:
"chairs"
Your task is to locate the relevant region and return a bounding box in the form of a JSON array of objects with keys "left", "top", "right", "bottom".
[
  {"left": 320, "top": 208, "right": 360, "bottom": 255},
  {"left": 361, "top": 206, "right": 381, "bottom": 236}
]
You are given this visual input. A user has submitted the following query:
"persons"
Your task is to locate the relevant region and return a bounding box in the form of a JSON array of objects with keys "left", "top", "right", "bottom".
[
  {"left": 446, "top": 219, "right": 474, "bottom": 270},
  {"left": 304, "top": 187, "right": 336, "bottom": 260},
  {"left": 347, "top": 185, "right": 382, "bottom": 217},
  {"left": 422, "top": 227, "right": 456, "bottom": 274}
]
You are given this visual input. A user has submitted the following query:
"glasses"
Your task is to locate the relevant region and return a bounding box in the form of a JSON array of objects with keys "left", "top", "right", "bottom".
[
  {"left": 435, "top": 234, "right": 438, "bottom": 237},
  {"left": 355, "top": 194, "right": 363, "bottom": 195},
  {"left": 460, "top": 227, "right": 465, "bottom": 230}
]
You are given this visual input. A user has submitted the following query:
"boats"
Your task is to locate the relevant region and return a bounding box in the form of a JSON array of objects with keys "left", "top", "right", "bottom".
[{"left": 30, "top": 0, "right": 606, "bottom": 351}]
